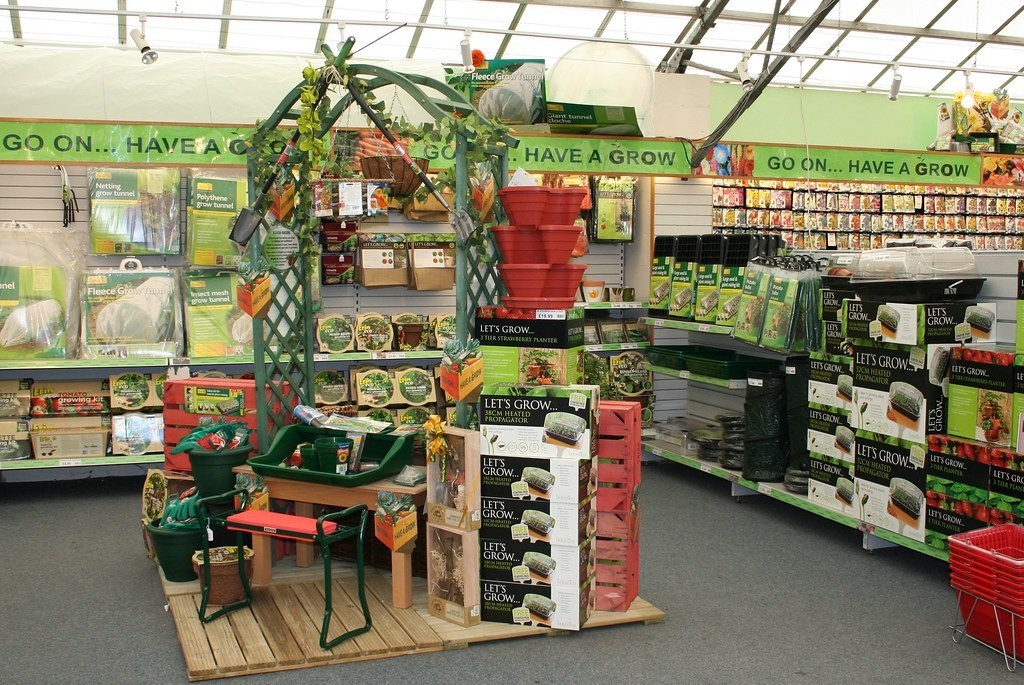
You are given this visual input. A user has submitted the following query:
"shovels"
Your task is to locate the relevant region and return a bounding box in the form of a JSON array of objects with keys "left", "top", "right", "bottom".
[{"left": 229, "top": 35, "right": 355, "bottom": 248}]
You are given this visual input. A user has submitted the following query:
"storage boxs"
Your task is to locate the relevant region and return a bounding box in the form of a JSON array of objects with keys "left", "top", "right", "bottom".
[
  {"left": 429, "top": 384, "right": 644, "bottom": 632},
  {"left": 162, "top": 379, "right": 299, "bottom": 473},
  {"left": 807, "top": 260, "right": 1024, "bottom": 551},
  {"left": 0, "top": 377, "right": 111, "bottom": 460},
  {"left": 948, "top": 521, "right": 1024, "bottom": 660},
  {"left": 475, "top": 305, "right": 585, "bottom": 387}
]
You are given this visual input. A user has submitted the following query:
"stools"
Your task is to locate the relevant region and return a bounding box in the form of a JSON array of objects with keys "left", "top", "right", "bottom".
[{"left": 195, "top": 490, "right": 371, "bottom": 648}]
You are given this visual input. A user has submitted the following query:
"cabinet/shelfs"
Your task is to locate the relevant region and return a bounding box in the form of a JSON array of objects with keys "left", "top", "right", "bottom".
[
  {"left": 638, "top": 315, "right": 747, "bottom": 497},
  {"left": 572, "top": 301, "right": 657, "bottom": 436},
  {"left": 0, "top": 342, "right": 444, "bottom": 482}
]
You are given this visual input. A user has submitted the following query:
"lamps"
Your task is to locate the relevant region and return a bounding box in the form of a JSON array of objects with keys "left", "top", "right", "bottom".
[
  {"left": 130, "top": 16, "right": 159, "bottom": 66},
  {"left": 736, "top": 51, "right": 753, "bottom": 92},
  {"left": 337, "top": 21, "right": 354, "bottom": 61},
  {"left": 889, "top": 64, "right": 903, "bottom": 102},
  {"left": 460, "top": 27, "right": 476, "bottom": 75},
  {"left": 961, "top": 70, "right": 975, "bottom": 109}
]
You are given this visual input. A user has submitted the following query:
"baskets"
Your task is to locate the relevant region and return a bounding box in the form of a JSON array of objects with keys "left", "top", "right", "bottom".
[{"left": 947, "top": 522, "right": 1024, "bottom": 660}]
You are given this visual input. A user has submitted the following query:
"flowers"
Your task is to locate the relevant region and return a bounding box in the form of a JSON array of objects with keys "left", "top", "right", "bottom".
[{"left": 955, "top": 89, "right": 994, "bottom": 132}]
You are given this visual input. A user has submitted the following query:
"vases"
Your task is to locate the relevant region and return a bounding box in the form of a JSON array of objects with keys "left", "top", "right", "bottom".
[
  {"left": 146, "top": 516, "right": 215, "bottom": 582},
  {"left": 392, "top": 324, "right": 424, "bottom": 350},
  {"left": 488, "top": 186, "right": 588, "bottom": 306},
  {"left": 194, "top": 545, "right": 254, "bottom": 606},
  {"left": 1000, "top": 143, "right": 1018, "bottom": 155},
  {"left": 181, "top": 444, "right": 254, "bottom": 505}
]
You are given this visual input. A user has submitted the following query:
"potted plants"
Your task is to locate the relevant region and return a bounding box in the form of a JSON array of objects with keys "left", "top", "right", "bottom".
[
  {"left": 520, "top": 350, "right": 556, "bottom": 385},
  {"left": 977, "top": 390, "right": 1010, "bottom": 443}
]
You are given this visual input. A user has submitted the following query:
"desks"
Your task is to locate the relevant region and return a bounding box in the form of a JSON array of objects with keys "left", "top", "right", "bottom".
[{"left": 231, "top": 455, "right": 427, "bottom": 608}]
